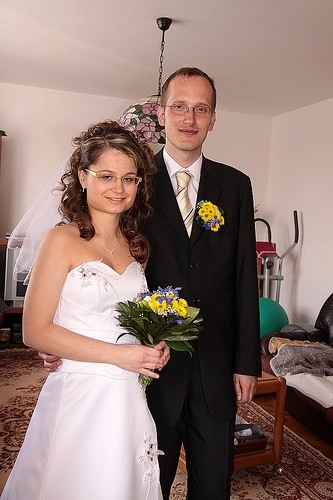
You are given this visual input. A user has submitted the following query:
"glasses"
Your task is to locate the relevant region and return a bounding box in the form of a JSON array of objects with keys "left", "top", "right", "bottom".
[
  {"left": 84, "top": 168, "right": 142, "bottom": 187},
  {"left": 162, "top": 104, "right": 215, "bottom": 118}
]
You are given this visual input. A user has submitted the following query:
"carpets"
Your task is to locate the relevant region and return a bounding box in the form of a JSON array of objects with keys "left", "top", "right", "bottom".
[{"left": 0, "top": 343, "right": 333, "bottom": 500}]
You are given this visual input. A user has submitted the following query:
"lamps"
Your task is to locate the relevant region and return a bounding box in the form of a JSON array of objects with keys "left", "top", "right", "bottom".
[{"left": 119, "top": 17, "right": 173, "bottom": 145}]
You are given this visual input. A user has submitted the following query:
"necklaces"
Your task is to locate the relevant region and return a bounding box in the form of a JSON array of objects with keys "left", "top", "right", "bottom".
[{"left": 94, "top": 230, "right": 121, "bottom": 258}]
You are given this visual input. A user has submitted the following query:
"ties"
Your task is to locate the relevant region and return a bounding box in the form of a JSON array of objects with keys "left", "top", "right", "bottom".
[{"left": 174, "top": 170, "right": 194, "bottom": 238}]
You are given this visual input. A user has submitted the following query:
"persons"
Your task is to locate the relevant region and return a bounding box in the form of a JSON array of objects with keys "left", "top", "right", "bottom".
[
  {"left": 117, "top": 67, "right": 262, "bottom": 500},
  {"left": 0, "top": 119, "right": 171, "bottom": 500}
]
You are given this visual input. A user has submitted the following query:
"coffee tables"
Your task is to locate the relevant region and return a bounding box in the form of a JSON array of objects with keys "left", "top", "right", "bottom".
[{"left": 171, "top": 371, "right": 286, "bottom": 482}]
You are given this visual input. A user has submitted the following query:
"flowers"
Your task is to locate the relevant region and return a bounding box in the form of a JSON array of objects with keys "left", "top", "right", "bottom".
[
  {"left": 117, "top": 285, "right": 203, "bottom": 388},
  {"left": 194, "top": 201, "right": 225, "bottom": 232}
]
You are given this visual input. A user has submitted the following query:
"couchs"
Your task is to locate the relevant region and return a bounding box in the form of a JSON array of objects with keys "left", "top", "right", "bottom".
[{"left": 261, "top": 293, "right": 333, "bottom": 446}]
